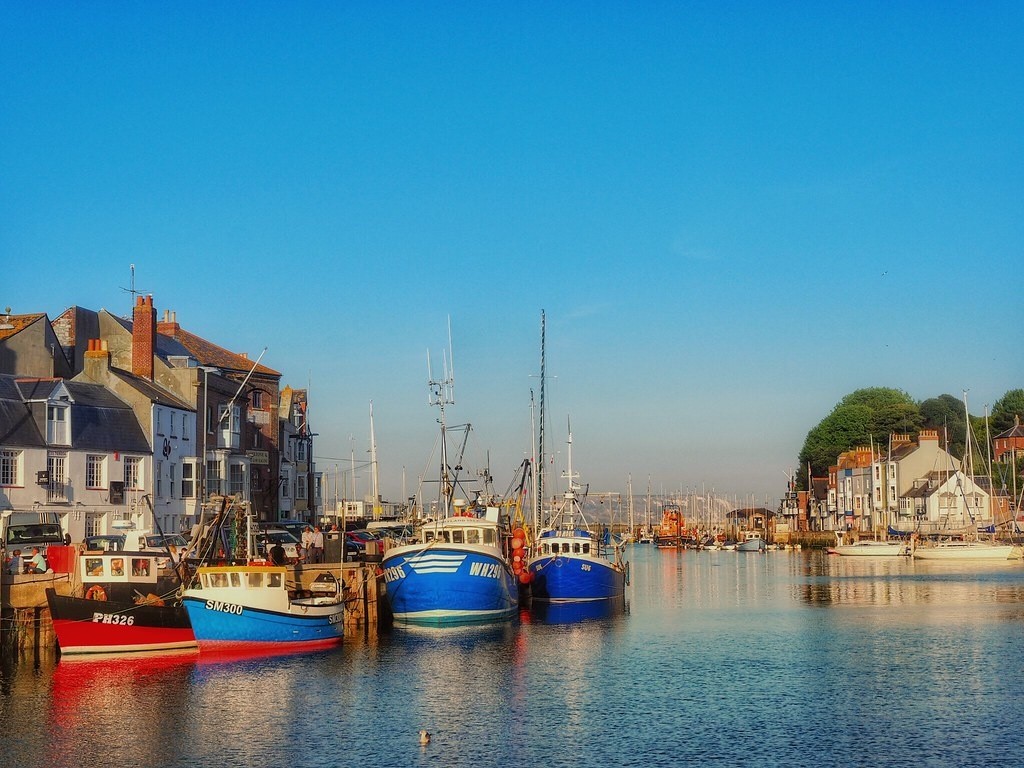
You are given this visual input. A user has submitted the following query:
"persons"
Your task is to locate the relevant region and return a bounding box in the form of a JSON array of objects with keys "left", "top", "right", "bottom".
[
  {"left": 328, "top": 524, "right": 339, "bottom": 540},
  {"left": 270, "top": 524, "right": 325, "bottom": 565},
  {"left": 9, "top": 547, "right": 47, "bottom": 574}
]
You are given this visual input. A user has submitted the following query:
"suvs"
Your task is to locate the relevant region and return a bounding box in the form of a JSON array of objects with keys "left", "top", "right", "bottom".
[
  {"left": 255, "top": 529, "right": 300, "bottom": 557},
  {"left": 138, "top": 534, "right": 194, "bottom": 566}
]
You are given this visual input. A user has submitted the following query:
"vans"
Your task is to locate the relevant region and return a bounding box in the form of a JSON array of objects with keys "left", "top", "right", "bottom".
[{"left": 260, "top": 521, "right": 315, "bottom": 542}]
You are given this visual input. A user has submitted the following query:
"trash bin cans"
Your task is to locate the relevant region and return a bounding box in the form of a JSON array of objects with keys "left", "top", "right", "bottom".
[{"left": 322, "top": 532, "right": 342, "bottom": 563}]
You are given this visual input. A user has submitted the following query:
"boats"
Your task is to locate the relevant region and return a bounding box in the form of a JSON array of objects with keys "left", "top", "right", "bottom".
[
  {"left": 528, "top": 412, "right": 630, "bottom": 602},
  {"left": 381, "top": 309, "right": 518, "bottom": 622},
  {"left": 46, "top": 485, "right": 197, "bottom": 654},
  {"left": 181, "top": 476, "right": 345, "bottom": 646}
]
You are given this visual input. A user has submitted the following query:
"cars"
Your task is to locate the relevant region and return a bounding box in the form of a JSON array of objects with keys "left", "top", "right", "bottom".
[
  {"left": 321, "top": 523, "right": 410, "bottom": 562},
  {"left": 80, "top": 535, "right": 125, "bottom": 552}
]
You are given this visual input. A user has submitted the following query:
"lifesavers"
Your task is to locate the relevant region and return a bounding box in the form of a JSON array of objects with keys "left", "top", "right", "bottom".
[{"left": 85, "top": 585, "right": 107, "bottom": 601}]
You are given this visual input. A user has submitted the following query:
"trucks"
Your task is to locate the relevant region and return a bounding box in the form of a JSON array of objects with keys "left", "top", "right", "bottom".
[{"left": 0, "top": 522, "right": 71, "bottom": 569}]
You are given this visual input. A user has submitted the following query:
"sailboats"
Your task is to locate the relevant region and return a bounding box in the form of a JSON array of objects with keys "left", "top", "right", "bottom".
[
  {"left": 913, "top": 389, "right": 1022, "bottom": 560},
  {"left": 618, "top": 467, "right": 801, "bottom": 551},
  {"left": 822, "top": 431, "right": 912, "bottom": 556}
]
